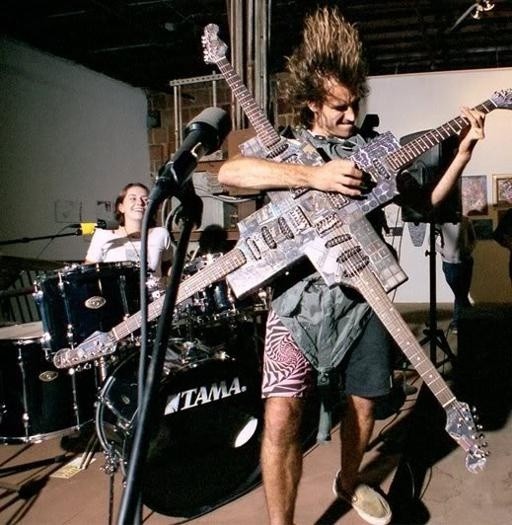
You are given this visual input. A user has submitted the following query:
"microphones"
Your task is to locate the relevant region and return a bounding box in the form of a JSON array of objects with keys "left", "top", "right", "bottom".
[
  {"left": 147, "top": 106, "right": 232, "bottom": 207},
  {"left": 68, "top": 218, "right": 122, "bottom": 231}
]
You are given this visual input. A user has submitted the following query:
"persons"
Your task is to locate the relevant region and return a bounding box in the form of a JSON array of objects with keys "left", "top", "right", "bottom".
[
  {"left": 433, "top": 209, "right": 476, "bottom": 335},
  {"left": 82, "top": 180, "right": 175, "bottom": 284},
  {"left": 218, "top": 61, "right": 484, "bottom": 525}
]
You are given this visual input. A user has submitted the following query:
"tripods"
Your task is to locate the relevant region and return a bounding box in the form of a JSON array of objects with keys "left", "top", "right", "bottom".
[{"left": 393, "top": 224, "right": 457, "bottom": 376}]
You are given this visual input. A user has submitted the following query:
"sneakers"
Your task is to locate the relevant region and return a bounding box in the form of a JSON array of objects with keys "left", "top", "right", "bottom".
[{"left": 333, "top": 470, "right": 392, "bottom": 525}]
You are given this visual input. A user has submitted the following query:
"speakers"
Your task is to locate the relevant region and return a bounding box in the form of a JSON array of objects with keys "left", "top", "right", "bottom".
[{"left": 393, "top": 128, "right": 464, "bottom": 223}]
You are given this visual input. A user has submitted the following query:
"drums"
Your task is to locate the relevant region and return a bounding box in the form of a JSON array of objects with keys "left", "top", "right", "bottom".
[
  {"left": 96, "top": 337, "right": 264, "bottom": 518},
  {"left": 174, "top": 253, "right": 262, "bottom": 328},
  {"left": 0, "top": 322, "right": 99, "bottom": 445},
  {"left": 32, "top": 260, "right": 149, "bottom": 354}
]
